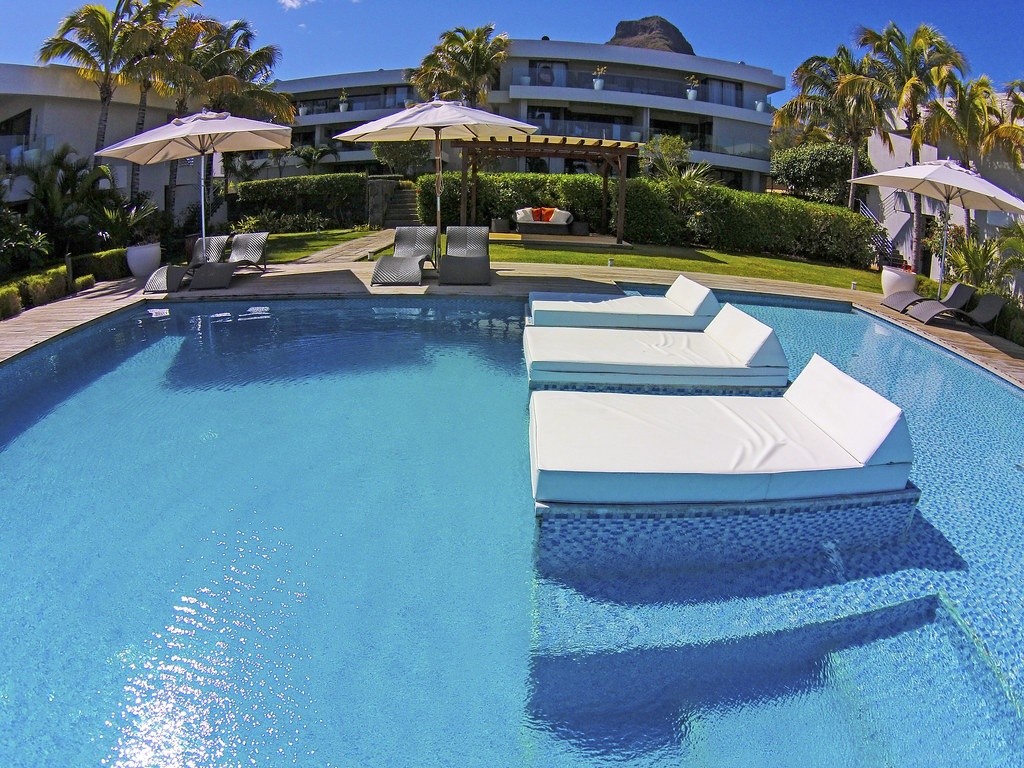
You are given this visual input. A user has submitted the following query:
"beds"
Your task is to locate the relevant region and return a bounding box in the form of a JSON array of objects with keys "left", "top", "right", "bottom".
[
  {"left": 522, "top": 301, "right": 787, "bottom": 380},
  {"left": 529, "top": 354, "right": 914, "bottom": 500},
  {"left": 528, "top": 275, "right": 720, "bottom": 326}
]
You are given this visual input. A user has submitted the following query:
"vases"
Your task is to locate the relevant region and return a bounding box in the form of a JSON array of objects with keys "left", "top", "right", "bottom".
[
  {"left": 519, "top": 75, "right": 531, "bottom": 85},
  {"left": 298, "top": 104, "right": 308, "bottom": 116},
  {"left": 630, "top": 131, "right": 643, "bottom": 141},
  {"left": 533, "top": 125, "right": 543, "bottom": 135},
  {"left": 755, "top": 100, "right": 765, "bottom": 112},
  {"left": 880, "top": 265, "right": 916, "bottom": 297}
]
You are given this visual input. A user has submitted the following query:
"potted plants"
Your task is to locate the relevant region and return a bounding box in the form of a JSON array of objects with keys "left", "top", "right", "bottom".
[
  {"left": 592, "top": 65, "right": 608, "bottom": 90},
  {"left": 335, "top": 88, "right": 352, "bottom": 112},
  {"left": 127, "top": 241, "right": 161, "bottom": 277}
]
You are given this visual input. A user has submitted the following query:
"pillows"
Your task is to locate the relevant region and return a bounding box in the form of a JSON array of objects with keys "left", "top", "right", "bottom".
[
  {"left": 532, "top": 208, "right": 541, "bottom": 221},
  {"left": 541, "top": 207, "right": 556, "bottom": 222},
  {"left": 549, "top": 208, "right": 571, "bottom": 224},
  {"left": 516, "top": 207, "right": 533, "bottom": 221}
]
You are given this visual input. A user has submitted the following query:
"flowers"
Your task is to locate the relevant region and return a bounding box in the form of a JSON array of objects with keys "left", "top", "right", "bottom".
[{"left": 897, "top": 264, "right": 915, "bottom": 274}]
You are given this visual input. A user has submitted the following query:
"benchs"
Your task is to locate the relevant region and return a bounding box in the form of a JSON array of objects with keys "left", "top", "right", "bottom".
[{"left": 512, "top": 212, "right": 573, "bottom": 234}]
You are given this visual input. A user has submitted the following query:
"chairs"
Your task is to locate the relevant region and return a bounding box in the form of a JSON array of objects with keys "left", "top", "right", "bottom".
[
  {"left": 143, "top": 235, "right": 232, "bottom": 294},
  {"left": 438, "top": 226, "right": 491, "bottom": 287},
  {"left": 880, "top": 281, "right": 978, "bottom": 313},
  {"left": 370, "top": 227, "right": 438, "bottom": 286},
  {"left": 189, "top": 231, "right": 271, "bottom": 291},
  {"left": 905, "top": 293, "right": 1011, "bottom": 327}
]
